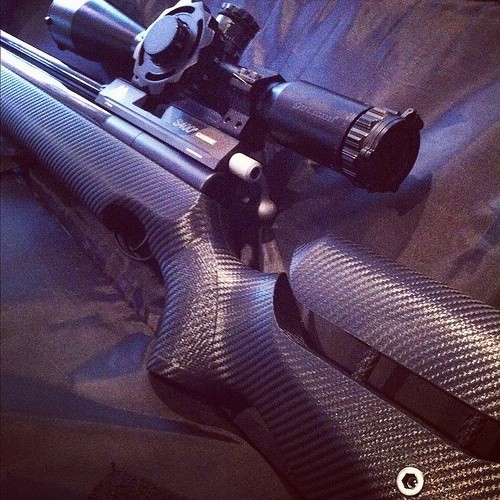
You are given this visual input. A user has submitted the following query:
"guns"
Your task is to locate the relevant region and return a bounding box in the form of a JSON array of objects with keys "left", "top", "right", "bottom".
[{"left": 0, "top": 0, "right": 500, "bottom": 499}]
